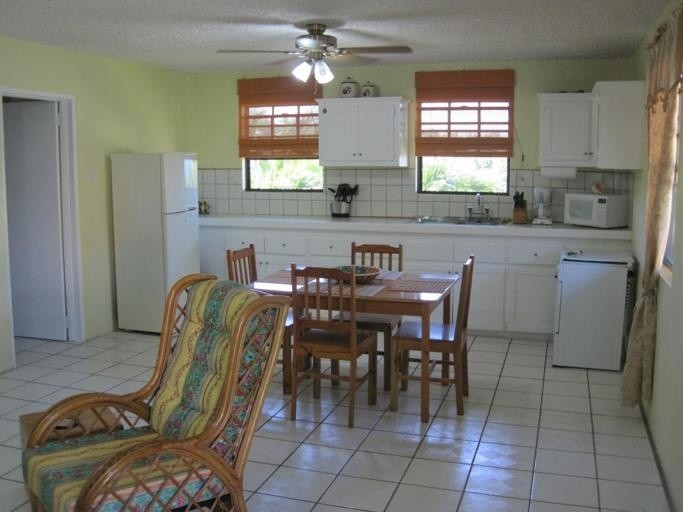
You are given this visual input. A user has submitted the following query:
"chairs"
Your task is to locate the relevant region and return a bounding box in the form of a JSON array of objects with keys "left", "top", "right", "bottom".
[
  {"left": 22, "top": 273, "right": 292, "bottom": 512},
  {"left": 226, "top": 243, "right": 305, "bottom": 396},
  {"left": 328, "top": 242, "right": 403, "bottom": 388},
  {"left": 286, "top": 261, "right": 381, "bottom": 426},
  {"left": 388, "top": 253, "right": 475, "bottom": 420}
]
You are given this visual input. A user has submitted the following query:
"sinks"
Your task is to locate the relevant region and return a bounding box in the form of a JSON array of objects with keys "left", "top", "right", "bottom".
[
  {"left": 417, "top": 216, "right": 468, "bottom": 225},
  {"left": 462, "top": 217, "right": 512, "bottom": 226}
]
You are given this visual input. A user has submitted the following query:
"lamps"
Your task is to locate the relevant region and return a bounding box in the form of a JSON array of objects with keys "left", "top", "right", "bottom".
[{"left": 291, "top": 49, "right": 335, "bottom": 85}]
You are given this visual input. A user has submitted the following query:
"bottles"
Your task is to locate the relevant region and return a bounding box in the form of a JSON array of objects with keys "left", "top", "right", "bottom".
[{"left": 339, "top": 75, "right": 376, "bottom": 98}]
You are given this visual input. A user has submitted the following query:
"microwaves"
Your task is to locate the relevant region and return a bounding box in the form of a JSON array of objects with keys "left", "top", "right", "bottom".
[{"left": 563, "top": 192, "right": 630, "bottom": 230}]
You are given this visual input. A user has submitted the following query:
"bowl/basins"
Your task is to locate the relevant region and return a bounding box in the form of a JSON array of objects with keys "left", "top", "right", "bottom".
[{"left": 332, "top": 265, "right": 382, "bottom": 284}]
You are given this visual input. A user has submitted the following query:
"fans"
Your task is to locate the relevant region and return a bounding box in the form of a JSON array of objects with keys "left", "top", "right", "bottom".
[{"left": 217, "top": 24, "right": 413, "bottom": 57}]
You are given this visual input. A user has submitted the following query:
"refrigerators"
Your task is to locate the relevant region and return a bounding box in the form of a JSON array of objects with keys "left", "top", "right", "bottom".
[{"left": 108, "top": 152, "right": 201, "bottom": 336}]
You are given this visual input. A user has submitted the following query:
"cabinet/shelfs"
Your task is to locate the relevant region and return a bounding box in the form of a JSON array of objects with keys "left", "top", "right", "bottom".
[
  {"left": 226, "top": 227, "right": 630, "bottom": 335},
  {"left": 314, "top": 96, "right": 410, "bottom": 168},
  {"left": 536, "top": 80, "right": 648, "bottom": 170}
]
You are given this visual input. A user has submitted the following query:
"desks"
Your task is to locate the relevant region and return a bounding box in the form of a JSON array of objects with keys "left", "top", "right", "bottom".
[{"left": 292, "top": 269, "right": 459, "bottom": 414}]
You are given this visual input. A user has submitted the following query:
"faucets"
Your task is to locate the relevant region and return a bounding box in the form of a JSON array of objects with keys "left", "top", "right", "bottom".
[{"left": 476, "top": 193, "right": 481, "bottom": 214}]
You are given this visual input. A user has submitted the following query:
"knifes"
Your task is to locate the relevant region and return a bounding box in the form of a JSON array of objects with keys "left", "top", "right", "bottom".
[{"left": 512, "top": 189, "right": 525, "bottom": 207}]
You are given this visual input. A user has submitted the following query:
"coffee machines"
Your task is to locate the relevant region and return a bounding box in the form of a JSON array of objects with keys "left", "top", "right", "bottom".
[{"left": 531, "top": 172, "right": 554, "bottom": 225}]
